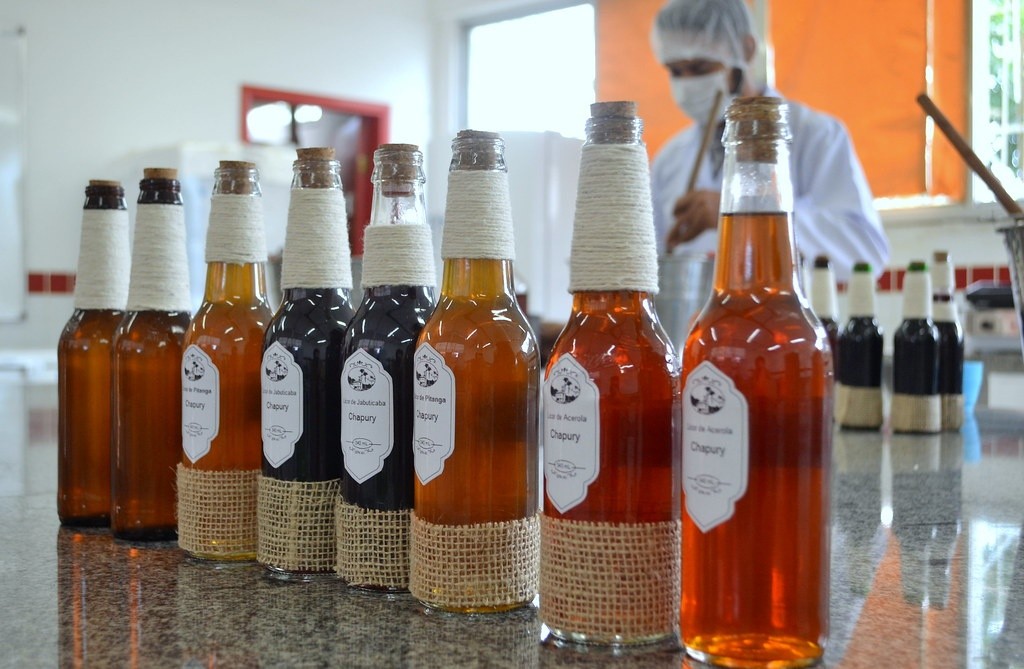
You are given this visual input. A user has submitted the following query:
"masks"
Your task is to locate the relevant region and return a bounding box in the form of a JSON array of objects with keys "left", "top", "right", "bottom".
[{"left": 673, "top": 64, "right": 744, "bottom": 127}]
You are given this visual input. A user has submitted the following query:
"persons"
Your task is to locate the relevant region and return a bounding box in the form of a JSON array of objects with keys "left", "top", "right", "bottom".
[{"left": 649, "top": 0, "right": 888, "bottom": 281}]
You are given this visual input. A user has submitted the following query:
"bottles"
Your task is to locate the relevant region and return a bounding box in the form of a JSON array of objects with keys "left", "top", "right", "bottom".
[
  {"left": 177, "top": 160, "right": 274, "bottom": 562},
  {"left": 836, "top": 260, "right": 887, "bottom": 431},
  {"left": 679, "top": 95, "right": 836, "bottom": 669},
  {"left": 807, "top": 256, "right": 838, "bottom": 344},
  {"left": 337, "top": 142, "right": 441, "bottom": 602},
  {"left": 929, "top": 250, "right": 965, "bottom": 433},
  {"left": 539, "top": 100, "right": 681, "bottom": 654},
  {"left": 411, "top": 128, "right": 539, "bottom": 611},
  {"left": 56, "top": 178, "right": 130, "bottom": 533},
  {"left": 892, "top": 260, "right": 942, "bottom": 435},
  {"left": 108, "top": 166, "right": 196, "bottom": 541},
  {"left": 256, "top": 146, "right": 355, "bottom": 573}
]
